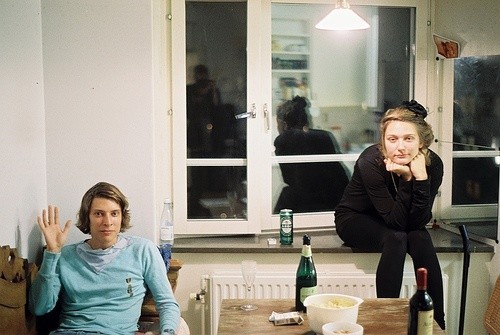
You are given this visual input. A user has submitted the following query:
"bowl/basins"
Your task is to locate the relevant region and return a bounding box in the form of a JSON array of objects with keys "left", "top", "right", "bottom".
[
  {"left": 321, "top": 322, "right": 364, "bottom": 335},
  {"left": 303, "top": 293, "right": 364, "bottom": 333}
]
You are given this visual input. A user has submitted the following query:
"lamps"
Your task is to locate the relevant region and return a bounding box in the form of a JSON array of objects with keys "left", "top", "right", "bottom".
[{"left": 314, "top": 0, "right": 370, "bottom": 31}]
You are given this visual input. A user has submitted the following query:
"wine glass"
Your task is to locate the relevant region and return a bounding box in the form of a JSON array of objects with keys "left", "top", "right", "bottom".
[{"left": 240, "top": 260, "right": 259, "bottom": 311}]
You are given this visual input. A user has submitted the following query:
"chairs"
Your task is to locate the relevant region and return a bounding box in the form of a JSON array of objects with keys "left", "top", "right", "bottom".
[
  {"left": 483, "top": 274, "right": 500, "bottom": 335},
  {"left": 0, "top": 244, "right": 50, "bottom": 335}
]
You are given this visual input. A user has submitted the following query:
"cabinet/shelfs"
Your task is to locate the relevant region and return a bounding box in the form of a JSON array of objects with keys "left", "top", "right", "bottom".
[{"left": 271, "top": 16, "right": 312, "bottom": 101}]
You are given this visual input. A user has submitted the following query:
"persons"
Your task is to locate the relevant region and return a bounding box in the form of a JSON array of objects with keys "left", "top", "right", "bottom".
[
  {"left": 29, "top": 181, "right": 181, "bottom": 335},
  {"left": 334, "top": 99, "right": 446, "bottom": 334},
  {"left": 186, "top": 64, "right": 222, "bottom": 194},
  {"left": 273, "top": 95, "right": 349, "bottom": 214}
]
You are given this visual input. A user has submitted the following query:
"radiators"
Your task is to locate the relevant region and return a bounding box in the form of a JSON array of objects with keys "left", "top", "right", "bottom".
[{"left": 189, "top": 269, "right": 449, "bottom": 335}]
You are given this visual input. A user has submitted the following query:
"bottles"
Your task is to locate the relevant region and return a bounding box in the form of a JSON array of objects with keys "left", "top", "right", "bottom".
[
  {"left": 295, "top": 234, "right": 317, "bottom": 313},
  {"left": 407, "top": 267, "right": 434, "bottom": 335},
  {"left": 158, "top": 199, "right": 175, "bottom": 248}
]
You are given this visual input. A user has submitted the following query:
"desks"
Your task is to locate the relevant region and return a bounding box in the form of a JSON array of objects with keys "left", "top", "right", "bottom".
[{"left": 217, "top": 298, "right": 446, "bottom": 335}]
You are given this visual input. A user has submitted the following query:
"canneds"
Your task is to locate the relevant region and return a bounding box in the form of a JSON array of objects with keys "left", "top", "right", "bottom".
[{"left": 279, "top": 208, "right": 293, "bottom": 245}]
